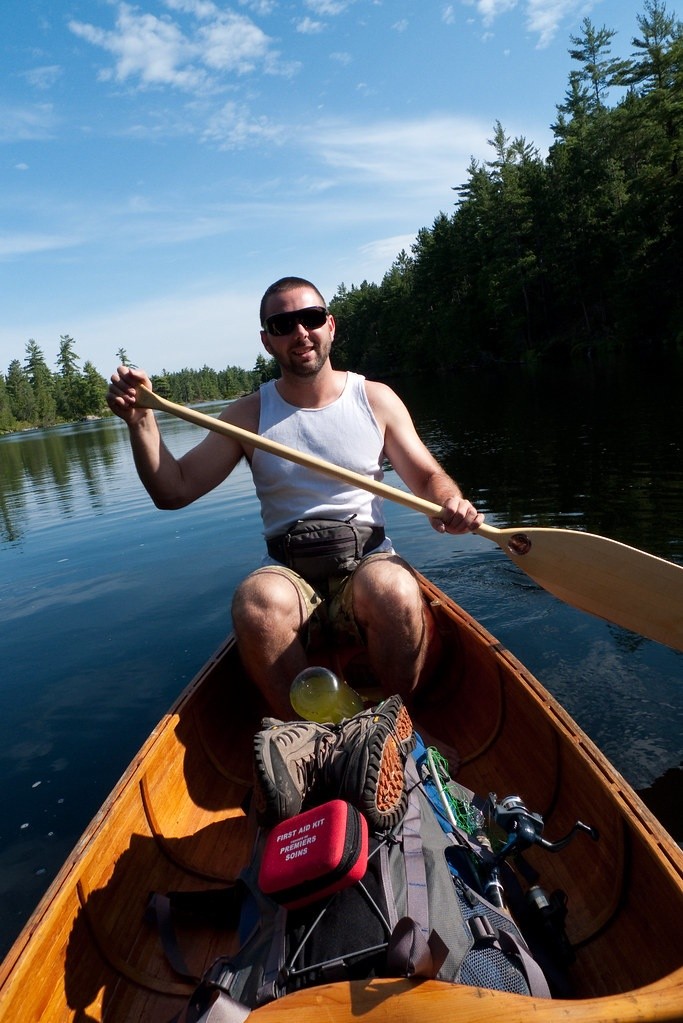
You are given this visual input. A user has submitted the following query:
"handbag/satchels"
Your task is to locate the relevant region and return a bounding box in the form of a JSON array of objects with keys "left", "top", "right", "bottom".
[{"left": 266, "top": 516, "right": 385, "bottom": 578}]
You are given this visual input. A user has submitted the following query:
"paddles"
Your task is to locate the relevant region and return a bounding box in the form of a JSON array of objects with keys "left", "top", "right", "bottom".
[{"left": 134, "top": 381, "right": 683, "bottom": 653}]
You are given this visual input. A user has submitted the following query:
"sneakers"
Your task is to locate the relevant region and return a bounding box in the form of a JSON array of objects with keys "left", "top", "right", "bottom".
[
  {"left": 250, "top": 717, "right": 337, "bottom": 828},
  {"left": 337, "top": 694, "right": 416, "bottom": 830}
]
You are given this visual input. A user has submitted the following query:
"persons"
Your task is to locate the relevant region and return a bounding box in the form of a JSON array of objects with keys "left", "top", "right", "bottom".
[{"left": 106, "top": 278, "right": 485, "bottom": 776}]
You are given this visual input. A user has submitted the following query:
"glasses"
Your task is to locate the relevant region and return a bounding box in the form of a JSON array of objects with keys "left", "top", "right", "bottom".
[{"left": 263, "top": 306, "right": 330, "bottom": 336}]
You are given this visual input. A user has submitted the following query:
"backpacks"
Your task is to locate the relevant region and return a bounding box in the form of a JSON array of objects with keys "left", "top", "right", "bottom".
[{"left": 221, "top": 734, "right": 554, "bottom": 1008}]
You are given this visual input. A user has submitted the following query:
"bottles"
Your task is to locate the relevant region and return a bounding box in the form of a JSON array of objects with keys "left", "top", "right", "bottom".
[{"left": 289, "top": 666, "right": 363, "bottom": 725}]
